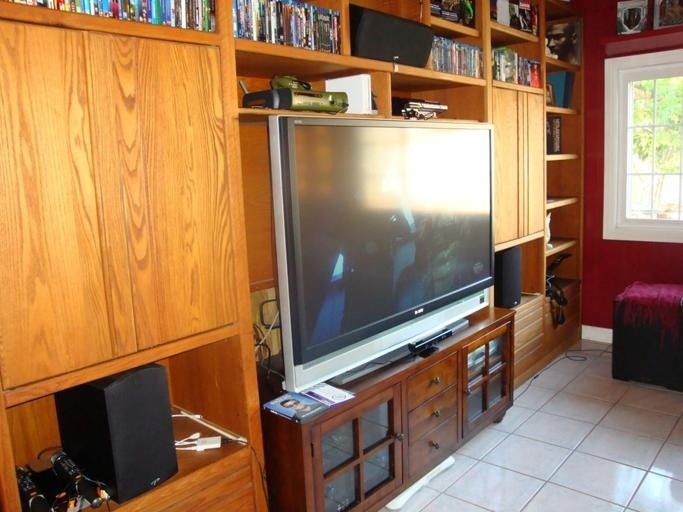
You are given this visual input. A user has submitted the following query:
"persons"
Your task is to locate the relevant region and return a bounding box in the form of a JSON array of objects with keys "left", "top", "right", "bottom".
[
  {"left": 280, "top": 399, "right": 320, "bottom": 420},
  {"left": 546, "top": 22, "right": 582, "bottom": 68}
]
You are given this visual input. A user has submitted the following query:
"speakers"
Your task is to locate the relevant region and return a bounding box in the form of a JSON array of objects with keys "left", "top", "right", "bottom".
[
  {"left": 494, "top": 246, "right": 521, "bottom": 308},
  {"left": 350, "top": 5, "right": 434, "bottom": 67},
  {"left": 54, "top": 362, "right": 179, "bottom": 504}
]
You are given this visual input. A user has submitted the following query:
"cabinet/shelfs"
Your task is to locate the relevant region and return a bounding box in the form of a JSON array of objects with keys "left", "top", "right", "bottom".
[
  {"left": 1, "top": 0, "right": 547, "bottom": 127},
  {"left": 404, "top": 344, "right": 462, "bottom": 484},
  {"left": 463, "top": 305, "right": 518, "bottom": 441},
  {"left": 544, "top": 3, "right": 587, "bottom": 354},
  {"left": 263, "top": 379, "right": 403, "bottom": 511},
  {"left": 0, "top": 13, "right": 245, "bottom": 390},
  {"left": 492, "top": 84, "right": 545, "bottom": 256},
  {"left": 80, "top": 408, "right": 264, "bottom": 512}
]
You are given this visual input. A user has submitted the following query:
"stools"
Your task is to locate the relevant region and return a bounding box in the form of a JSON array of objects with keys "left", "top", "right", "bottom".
[{"left": 608, "top": 280, "right": 683, "bottom": 396}]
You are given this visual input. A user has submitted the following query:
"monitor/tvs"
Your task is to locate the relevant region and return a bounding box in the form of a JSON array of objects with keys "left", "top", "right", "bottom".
[{"left": 266, "top": 113, "right": 494, "bottom": 394}]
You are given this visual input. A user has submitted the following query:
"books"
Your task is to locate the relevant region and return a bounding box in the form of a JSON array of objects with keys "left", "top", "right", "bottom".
[
  {"left": 233, "top": 0, "right": 339, "bottom": 55},
  {"left": 404, "top": 30, "right": 543, "bottom": 90},
  {"left": 12, "top": 0, "right": 216, "bottom": 33},
  {"left": 300, "top": 383, "right": 356, "bottom": 407},
  {"left": 263, "top": 391, "right": 329, "bottom": 424}
]
